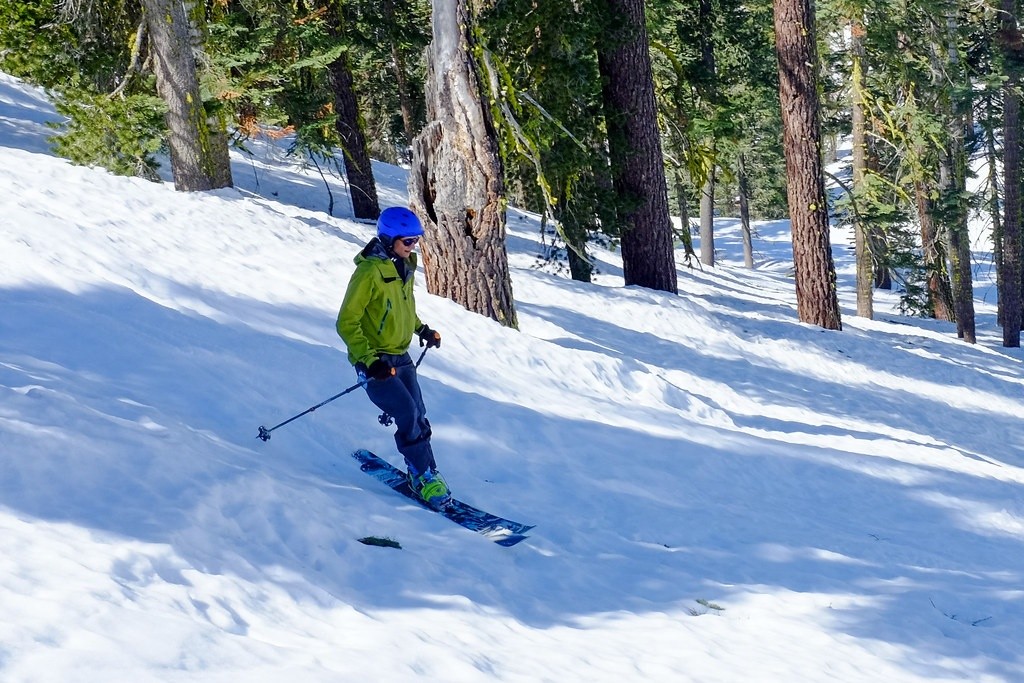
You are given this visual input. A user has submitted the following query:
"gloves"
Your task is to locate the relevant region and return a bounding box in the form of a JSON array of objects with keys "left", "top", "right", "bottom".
[
  {"left": 368, "top": 359, "right": 392, "bottom": 379},
  {"left": 416, "top": 324, "right": 441, "bottom": 347}
]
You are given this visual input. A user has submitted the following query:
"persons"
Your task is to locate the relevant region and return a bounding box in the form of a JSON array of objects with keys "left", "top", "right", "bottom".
[{"left": 335, "top": 207, "right": 453, "bottom": 513}]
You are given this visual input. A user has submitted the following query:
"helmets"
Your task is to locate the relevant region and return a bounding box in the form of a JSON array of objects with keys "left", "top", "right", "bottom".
[{"left": 376, "top": 207, "right": 424, "bottom": 248}]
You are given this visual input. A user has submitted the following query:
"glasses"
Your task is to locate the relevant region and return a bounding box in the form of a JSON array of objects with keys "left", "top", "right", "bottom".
[{"left": 399, "top": 237, "right": 419, "bottom": 246}]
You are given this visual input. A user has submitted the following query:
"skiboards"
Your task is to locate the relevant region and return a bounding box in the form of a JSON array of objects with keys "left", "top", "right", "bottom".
[{"left": 349, "top": 446, "right": 537, "bottom": 548}]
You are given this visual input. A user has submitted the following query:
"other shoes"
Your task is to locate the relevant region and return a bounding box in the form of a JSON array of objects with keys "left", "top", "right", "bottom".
[{"left": 406, "top": 471, "right": 451, "bottom": 508}]
